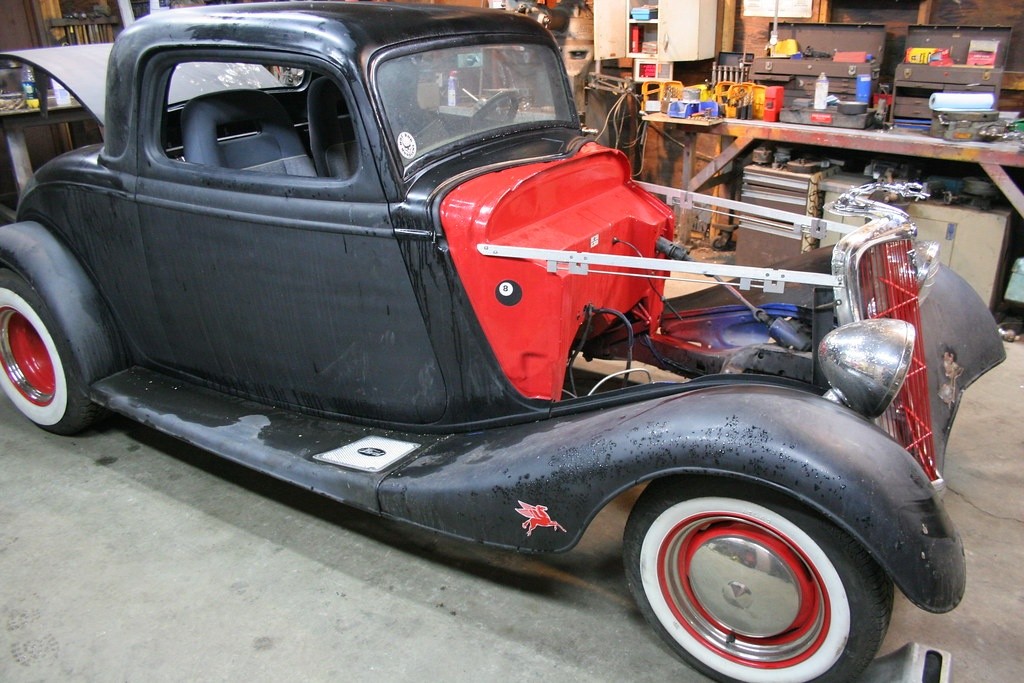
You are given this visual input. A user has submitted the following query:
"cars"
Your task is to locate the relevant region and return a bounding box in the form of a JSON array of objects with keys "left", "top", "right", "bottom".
[{"left": 0, "top": 0, "right": 1009, "bottom": 683}]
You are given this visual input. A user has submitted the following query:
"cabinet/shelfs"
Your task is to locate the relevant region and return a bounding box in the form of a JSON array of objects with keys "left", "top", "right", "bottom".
[{"left": 594, "top": 0, "right": 717, "bottom": 64}]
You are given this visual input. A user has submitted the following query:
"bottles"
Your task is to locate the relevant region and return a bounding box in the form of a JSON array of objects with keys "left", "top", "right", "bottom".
[
  {"left": 20, "top": 63, "right": 40, "bottom": 110},
  {"left": 814, "top": 72, "right": 828, "bottom": 110},
  {"left": 661, "top": 85, "right": 672, "bottom": 113},
  {"left": 728, "top": 86, "right": 754, "bottom": 119},
  {"left": 929, "top": 92, "right": 994, "bottom": 111},
  {"left": 631, "top": 25, "right": 639, "bottom": 52}
]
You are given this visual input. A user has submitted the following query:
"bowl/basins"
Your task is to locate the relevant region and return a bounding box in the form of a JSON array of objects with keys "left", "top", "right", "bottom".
[{"left": 837, "top": 100, "right": 868, "bottom": 115}]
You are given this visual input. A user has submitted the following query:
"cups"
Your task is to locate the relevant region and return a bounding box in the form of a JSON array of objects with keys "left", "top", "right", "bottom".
[
  {"left": 50, "top": 79, "right": 71, "bottom": 107},
  {"left": 682, "top": 86, "right": 701, "bottom": 102},
  {"left": 857, "top": 64, "right": 871, "bottom": 102}
]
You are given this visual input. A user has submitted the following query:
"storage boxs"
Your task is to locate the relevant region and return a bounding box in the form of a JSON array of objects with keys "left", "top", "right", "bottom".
[{"left": 630, "top": 8, "right": 658, "bottom": 20}]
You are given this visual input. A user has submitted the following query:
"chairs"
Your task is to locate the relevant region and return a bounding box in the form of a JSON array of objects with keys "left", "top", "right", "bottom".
[
  {"left": 308, "top": 76, "right": 394, "bottom": 180},
  {"left": 185, "top": 88, "right": 317, "bottom": 178}
]
